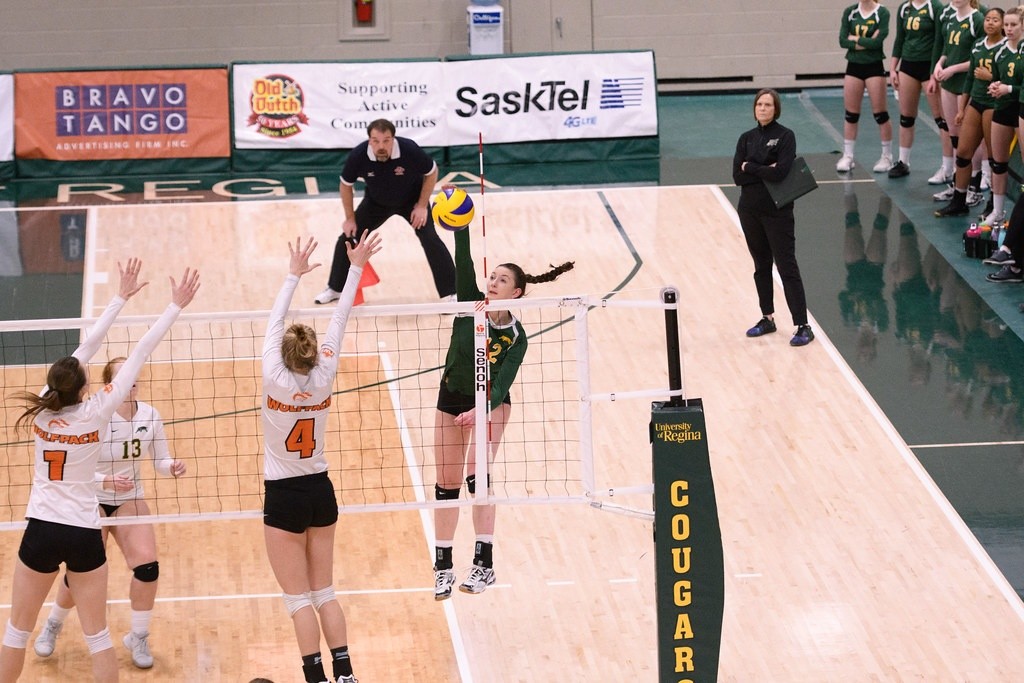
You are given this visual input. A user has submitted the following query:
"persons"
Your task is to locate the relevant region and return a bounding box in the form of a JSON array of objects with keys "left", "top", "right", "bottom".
[
  {"left": 732, "top": 87, "right": 815, "bottom": 347},
  {"left": 0, "top": 258, "right": 201, "bottom": 683},
  {"left": 888, "top": 0, "right": 944, "bottom": 179},
  {"left": 836, "top": 0, "right": 894, "bottom": 171},
  {"left": 262, "top": 229, "right": 382, "bottom": 683},
  {"left": 33, "top": 356, "right": 187, "bottom": 668},
  {"left": 432, "top": 184, "right": 579, "bottom": 601},
  {"left": 926, "top": 0, "right": 1024, "bottom": 284},
  {"left": 315, "top": 119, "right": 456, "bottom": 303},
  {"left": 837, "top": 171, "right": 1024, "bottom": 437}
]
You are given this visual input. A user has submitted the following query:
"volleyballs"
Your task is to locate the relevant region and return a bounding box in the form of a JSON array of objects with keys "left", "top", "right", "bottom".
[{"left": 430, "top": 188, "right": 476, "bottom": 231}]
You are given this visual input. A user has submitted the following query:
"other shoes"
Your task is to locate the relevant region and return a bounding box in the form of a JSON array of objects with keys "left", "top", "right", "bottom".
[{"left": 336, "top": 673, "right": 358, "bottom": 683}]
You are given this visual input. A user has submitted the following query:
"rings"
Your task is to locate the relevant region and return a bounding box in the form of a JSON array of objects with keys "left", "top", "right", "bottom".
[{"left": 420, "top": 219, "right": 423, "bottom": 221}]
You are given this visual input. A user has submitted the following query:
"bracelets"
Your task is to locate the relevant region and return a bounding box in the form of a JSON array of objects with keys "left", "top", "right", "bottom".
[{"left": 1008, "top": 85, "right": 1012, "bottom": 93}]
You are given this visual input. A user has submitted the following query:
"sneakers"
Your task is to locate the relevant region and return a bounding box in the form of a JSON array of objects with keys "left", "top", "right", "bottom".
[
  {"left": 986, "top": 265, "right": 1023, "bottom": 283},
  {"left": 459, "top": 564, "right": 496, "bottom": 594},
  {"left": 790, "top": 324, "right": 814, "bottom": 345},
  {"left": 931, "top": 184, "right": 974, "bottom": 205},
  {"left": 966, "top": 190, "right": 984, "bottom": 206},
  {"left": 123, "top": 632, "right": 154, "bottom": 668},
  {"left": 441, "top": 295, "right": 456, "bottom": 302},
  {"left": 746, "top": 316, "right": 776, "bottom": 336},
  {"left": 434, "top": 568, "right": 456, "bottom": 601},
  {"left": 980, "top": 176, "right": 992, "bottom": 190},
  {"left": 928, "top": 168, "right": 953, "bottom": 184},
  {"left": 34, "top": 617, "right": 63, "bottom": 658},
  {"left": 983, "top": 248, "right": 1015, "bottom": 265},
  {"left": 836, "top": 155, "right": 855, "bottom": 171},
  {"left": 315, "top": 287, "right": 341, "bottom": 304},
  {"left": 934, "top": 202, "right": 969, "bottom": 217},
  {"left": 887, "top": 161, "right": 910, "bottom": 178},
  {"left": 873, "top": 156, "right": 892, "bottom": 172}
]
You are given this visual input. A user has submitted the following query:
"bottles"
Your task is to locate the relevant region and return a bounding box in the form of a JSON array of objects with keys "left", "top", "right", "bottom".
[{"left": 966, "top": 222, "right": 1006, "bottom": 248}]
